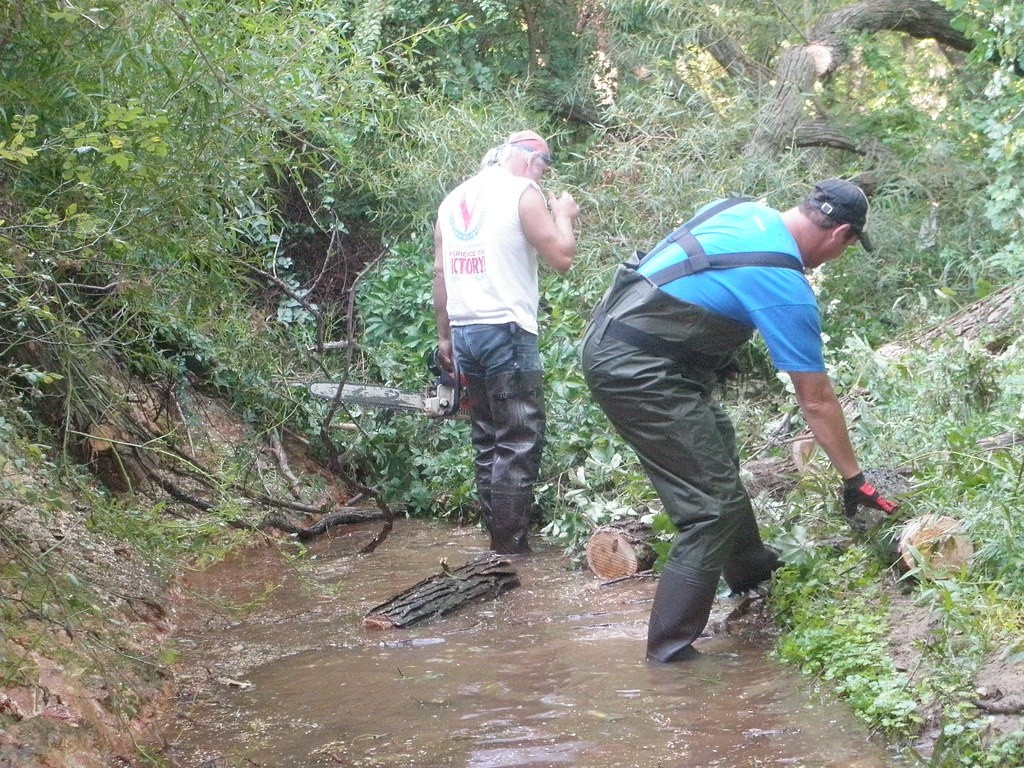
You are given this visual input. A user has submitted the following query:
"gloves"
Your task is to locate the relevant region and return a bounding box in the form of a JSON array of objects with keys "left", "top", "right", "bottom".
[
  {"left": 716, "top": 358, "right": 743, "bottom": 386},
  {"left": 841, "top": 472, "right": 901, "bottom": 518}
]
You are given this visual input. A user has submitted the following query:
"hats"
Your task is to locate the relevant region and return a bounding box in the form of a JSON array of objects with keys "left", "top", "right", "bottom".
[
  {"left": 505, "top": 130, "right": 552, "bottom": 166},
  {"left": 807, "top": 180, "right": 872, "bottom": 253}
]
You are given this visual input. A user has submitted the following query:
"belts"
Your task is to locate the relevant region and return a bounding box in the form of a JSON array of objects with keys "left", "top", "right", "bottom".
[{"left": 592, "top": 306, "right": 728, "bottom": 371}]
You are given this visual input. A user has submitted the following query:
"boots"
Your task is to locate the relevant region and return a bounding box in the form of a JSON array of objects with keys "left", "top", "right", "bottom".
[
  {"left": 490, "top": 491, "right": 533, "bottom": 554},
  {"left": 476, "top": 484, "right": 495, "bottom": 550},
  {"left": 722, "top": 506, "right": 786, "bottom": 594},
  {"left": 645, "top": 558, "right": 720, "bottom": 663}
]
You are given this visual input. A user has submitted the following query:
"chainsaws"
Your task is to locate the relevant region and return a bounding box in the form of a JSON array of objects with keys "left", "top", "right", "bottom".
[{"left": 308, "top": 345, "right": 470, "bottom": 421}]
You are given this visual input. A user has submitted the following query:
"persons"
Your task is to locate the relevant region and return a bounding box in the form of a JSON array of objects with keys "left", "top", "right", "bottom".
[
  {"left": 583, "top": 179, "right": 898, "bottom": 663},
  {"left": 434, "top": 130, "right": 581, "bottom": 554}
]
